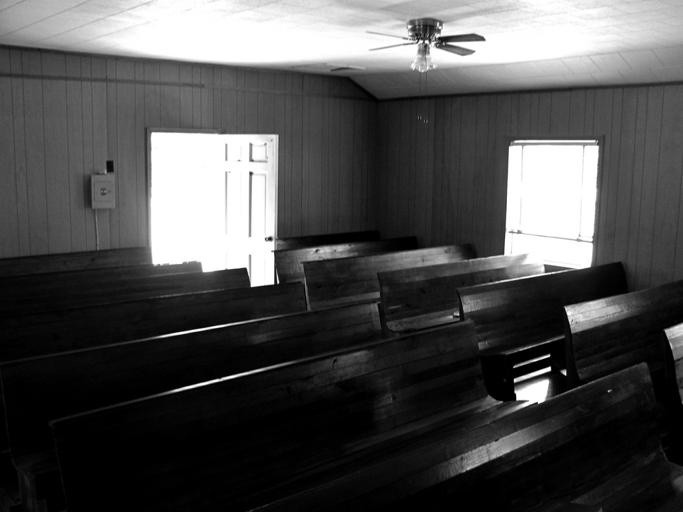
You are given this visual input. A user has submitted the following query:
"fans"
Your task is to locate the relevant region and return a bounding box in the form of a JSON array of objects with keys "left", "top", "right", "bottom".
[{"left": 365, "top": 19, "right": 485, "bottom": 56}]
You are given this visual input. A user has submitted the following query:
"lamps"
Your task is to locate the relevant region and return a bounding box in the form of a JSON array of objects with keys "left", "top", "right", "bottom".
[{"left": 410, "top": 43, "right": 438, "bottom": 128}]
